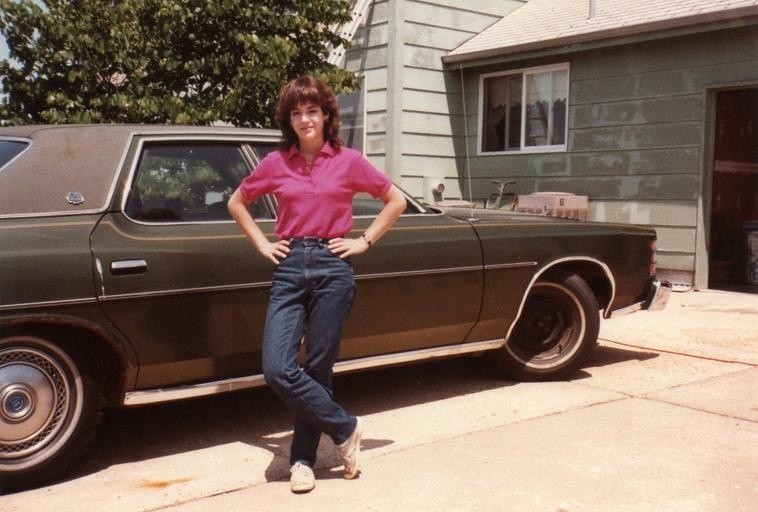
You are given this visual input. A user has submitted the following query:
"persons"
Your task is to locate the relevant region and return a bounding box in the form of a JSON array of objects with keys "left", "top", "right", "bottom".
[
  {"left": 223, "top": 74, "right": 409, "bottom": 493},
  {"left": 494, "top": 85, "right": 557, "bottom": 146}
]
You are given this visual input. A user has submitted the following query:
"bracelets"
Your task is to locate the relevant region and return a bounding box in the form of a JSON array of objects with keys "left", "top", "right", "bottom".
[{"left": 362, "top": 232, "right": 372, "bottom": 248}]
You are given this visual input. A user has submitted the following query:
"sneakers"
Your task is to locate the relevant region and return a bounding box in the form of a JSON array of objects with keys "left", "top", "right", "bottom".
[
  {"left": 290, "top": 462, "right": 317, "bottom": 492},
  {"left": 335, "top": 415, "right": 363, "bottom": 480}
]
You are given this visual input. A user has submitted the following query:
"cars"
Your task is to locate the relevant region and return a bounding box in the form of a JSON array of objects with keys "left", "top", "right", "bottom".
[{"left": 0, "top": 120, "right": 673, "bottom": 496}]
